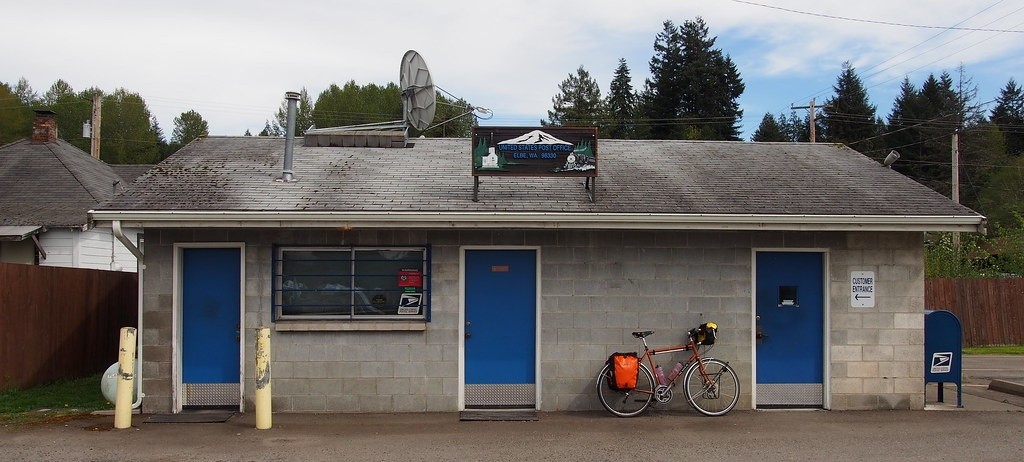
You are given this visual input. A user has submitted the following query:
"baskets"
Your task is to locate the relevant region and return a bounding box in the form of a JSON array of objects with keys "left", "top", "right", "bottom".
[{"left": 695, "top": 328, "right": 719, "bottom": 346}]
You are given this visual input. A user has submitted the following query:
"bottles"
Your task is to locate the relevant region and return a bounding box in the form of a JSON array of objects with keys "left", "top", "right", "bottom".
[
  {"left": 655, "top": 364, "right": 666, "bottom": 385},
  {"left": 667, "top": 361, "right": 683, "bottom": 381}
]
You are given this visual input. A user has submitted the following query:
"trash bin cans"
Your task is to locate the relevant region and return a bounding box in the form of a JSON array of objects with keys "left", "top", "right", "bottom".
[{"left": 923, "top": 309, "right": 963, "bottom": 409}]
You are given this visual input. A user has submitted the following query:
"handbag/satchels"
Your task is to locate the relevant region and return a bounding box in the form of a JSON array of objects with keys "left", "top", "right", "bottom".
[{"left": 607, "top": 353, "right": 639, "bottom": 391}]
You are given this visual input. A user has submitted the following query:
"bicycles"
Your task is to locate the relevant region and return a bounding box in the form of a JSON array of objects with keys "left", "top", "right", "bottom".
[{"left": 597, "top": 326, "right": 741, "bottom": 418}]
